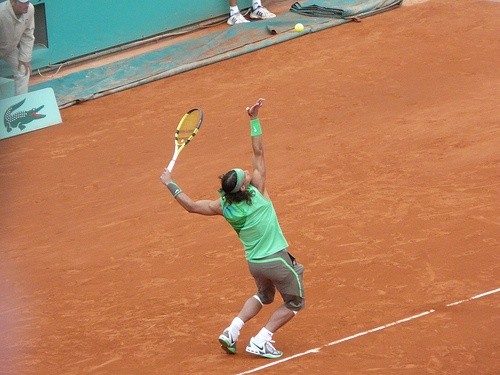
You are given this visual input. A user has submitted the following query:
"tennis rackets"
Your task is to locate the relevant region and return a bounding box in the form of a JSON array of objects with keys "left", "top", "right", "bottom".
[{"left": 167, "top": 106, "right": 203, "bottom": 172}]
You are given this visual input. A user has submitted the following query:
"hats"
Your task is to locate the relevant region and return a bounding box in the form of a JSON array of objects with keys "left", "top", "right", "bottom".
[{"left": 18, "top": 0, "right": 29, "bottom": 3}]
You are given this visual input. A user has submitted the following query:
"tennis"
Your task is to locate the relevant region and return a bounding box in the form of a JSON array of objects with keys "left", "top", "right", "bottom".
[{"left": 295, "top": 22, "right": 304, "bottom": 33}]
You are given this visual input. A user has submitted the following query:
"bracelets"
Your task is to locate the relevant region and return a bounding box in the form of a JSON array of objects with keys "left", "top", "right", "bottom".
[
  {"left": 167, "top": 181, "right": 183, "bottom": 199},
  {"left": 250, "top": 118, "right": 262, "bottom": 136}
]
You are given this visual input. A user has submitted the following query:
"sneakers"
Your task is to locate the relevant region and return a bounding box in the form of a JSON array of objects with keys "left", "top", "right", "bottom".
[
  {"left": 218, "top": 328, "right": 236, "bottom": 354},
  {"left": 227, "top": 12, "right": 250, "bottom": 25},
  {"left": 245, "top": 337, "right": 283, "bottom": 359},
  {"left": 250, "top": 6, "right": 276, "bottom": 18}
]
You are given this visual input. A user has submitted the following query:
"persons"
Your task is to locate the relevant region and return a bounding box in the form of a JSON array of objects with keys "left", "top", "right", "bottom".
[
  {"left": 160, "top": 98, "right": 304, "bottom": 358},
  {"left": 0, "top": 0, "right": 35, "bottom": 95},
  {"left": 227, "top": 0, "right": 276, "bottom": 25}
]
